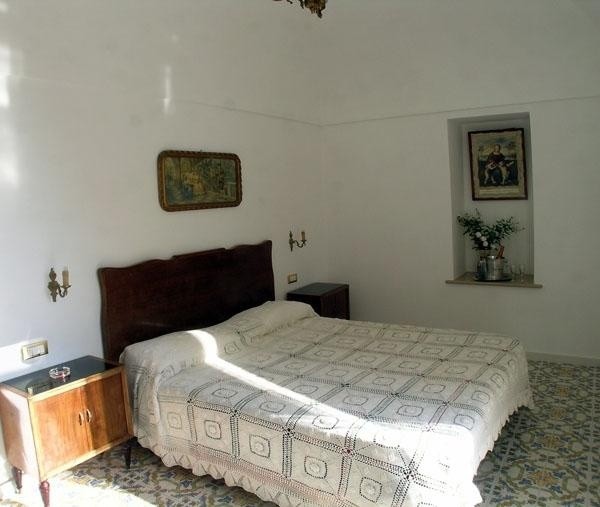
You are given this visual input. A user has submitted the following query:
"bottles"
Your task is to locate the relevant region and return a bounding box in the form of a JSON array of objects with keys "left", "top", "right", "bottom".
[
  {"left": 495, "top": 245, "right": 504, "bottom": 258},
  {"left": 476, "top": 255, "right": 486, "bottom": 280}
]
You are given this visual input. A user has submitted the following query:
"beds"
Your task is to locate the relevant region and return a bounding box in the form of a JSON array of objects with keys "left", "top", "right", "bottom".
[{"left": 94, "top": 239, "right": 535, "bottom": 506}]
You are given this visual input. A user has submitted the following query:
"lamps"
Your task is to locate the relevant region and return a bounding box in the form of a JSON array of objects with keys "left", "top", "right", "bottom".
[
  {"left": 48, "top": 262, "right": 75, "bottom": 302},
  {"left": 287, "top": 228, "right": 312, "bottom": 250}
]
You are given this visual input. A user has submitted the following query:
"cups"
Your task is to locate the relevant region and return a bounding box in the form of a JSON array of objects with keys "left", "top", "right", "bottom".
[
  {"left": 487, "top": 255, "right": 504, "bottom": 280},
  {"left": 509, "top": 263, "right": 525, "bottom": 283}
]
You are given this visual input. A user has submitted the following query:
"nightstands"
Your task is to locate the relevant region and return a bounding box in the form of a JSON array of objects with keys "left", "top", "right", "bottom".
[
  {"left": 0, "top": 352, "right": 138, "bottom": 507},
  {"left": 285, "top": 278, "right": 350, "bottom": 321}
]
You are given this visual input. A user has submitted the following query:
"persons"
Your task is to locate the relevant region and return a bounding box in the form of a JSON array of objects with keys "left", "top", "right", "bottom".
[{"left": 484, "top": 143, "right": 507, "bottom": 186}]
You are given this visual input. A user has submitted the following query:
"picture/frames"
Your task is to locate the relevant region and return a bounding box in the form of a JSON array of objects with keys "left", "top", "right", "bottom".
[
  {"left": 156, "top": 147, "right": 242, "bottom": 212},
  {"left": 468, "top": 125, "right": 529, "bottom": 202},
  {"left": 475, "top": 250, "right": 505, "bottom": 282}
]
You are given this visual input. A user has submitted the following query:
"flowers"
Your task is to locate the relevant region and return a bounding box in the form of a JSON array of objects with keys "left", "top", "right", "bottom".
[{"left": 456, "top": 207, "right": 529, "bottom": 250}]
